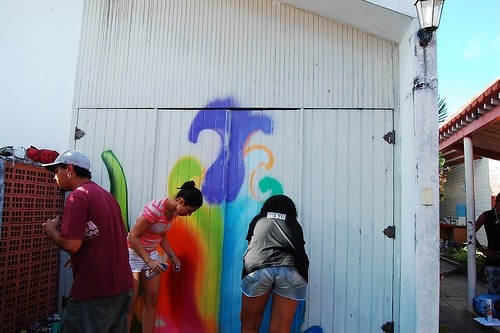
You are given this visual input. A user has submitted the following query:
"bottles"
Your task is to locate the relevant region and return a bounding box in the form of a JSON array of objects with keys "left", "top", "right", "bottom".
[
  {"left": 22, "top": 312, "right": 61, "bottom": 333},
  {"left": 486, "top": 298, "right": 493, "bottom": 322},
  {"left": 145, "top": 263, "right": 169, "bottom": 278}
]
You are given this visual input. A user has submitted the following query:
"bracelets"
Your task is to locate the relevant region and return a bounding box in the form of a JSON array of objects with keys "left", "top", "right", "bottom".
[{"left": 168, "top": 252, "right": 175, "bottom": 258}]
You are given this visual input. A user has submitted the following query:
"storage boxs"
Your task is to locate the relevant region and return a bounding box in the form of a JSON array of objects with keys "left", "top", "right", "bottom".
[{"left": 473, "top": 293, "right": 500, "bottom": 316}]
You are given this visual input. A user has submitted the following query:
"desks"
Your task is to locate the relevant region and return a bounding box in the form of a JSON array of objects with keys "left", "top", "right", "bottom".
[{"left": 440, "top": 224, "right": 467, "bottom": 242}]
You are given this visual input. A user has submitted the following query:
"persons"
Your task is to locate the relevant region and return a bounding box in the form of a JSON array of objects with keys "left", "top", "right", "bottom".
[
  {"left": 41, "top": 149, "right": 135, "bottom": 332},
  {"left": 240, "top": 194, "right": 309, "bottom": 333},
  {"left": 126, "top": 179, "right": 202, "bottom": 332},
  {"left": 475, "top": 191, "right": 500, "bottom": 296}
]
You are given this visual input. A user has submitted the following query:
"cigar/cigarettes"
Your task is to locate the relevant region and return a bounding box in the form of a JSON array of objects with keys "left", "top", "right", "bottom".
[{"left": 42, "top": 218, "right": 55, "bottom": 225}]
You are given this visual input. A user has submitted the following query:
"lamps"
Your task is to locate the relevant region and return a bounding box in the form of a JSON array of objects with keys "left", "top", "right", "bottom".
[{"left": 414, "top": 0, "right": 445, "bottom": 46}]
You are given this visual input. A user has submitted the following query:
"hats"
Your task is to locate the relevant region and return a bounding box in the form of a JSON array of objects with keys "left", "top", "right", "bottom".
[{"left": 42, "top": 149, "right": 91, "bottom": 172}]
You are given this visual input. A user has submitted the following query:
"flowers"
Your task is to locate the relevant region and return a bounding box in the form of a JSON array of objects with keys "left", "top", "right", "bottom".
[{"left": 439, "top": 152, "right": 452, "bottom": 203}]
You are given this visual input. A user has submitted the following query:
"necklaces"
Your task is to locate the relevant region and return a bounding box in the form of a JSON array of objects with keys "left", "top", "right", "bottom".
[{"left": 494, "top": 206, "right": 500, "bottom": 226}]
